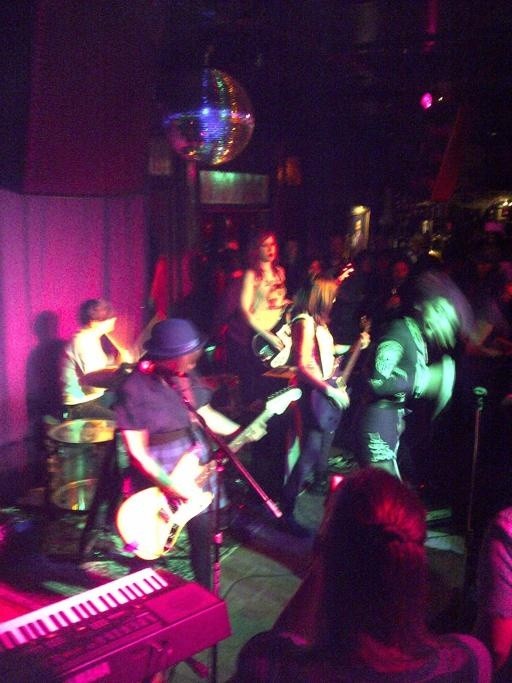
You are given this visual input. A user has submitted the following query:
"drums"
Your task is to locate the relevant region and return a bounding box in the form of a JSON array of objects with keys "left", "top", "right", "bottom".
[
  {"left": 195, "top": 346, "right": 239, "bottom": 392},
  {"left": 48, "top": 418, "right": 117, "bottom": 514}
]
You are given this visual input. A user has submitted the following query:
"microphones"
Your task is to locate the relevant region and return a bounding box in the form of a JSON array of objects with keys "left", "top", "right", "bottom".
[{"left": 139, "top": 360, "right": 190, "bottom": 375}]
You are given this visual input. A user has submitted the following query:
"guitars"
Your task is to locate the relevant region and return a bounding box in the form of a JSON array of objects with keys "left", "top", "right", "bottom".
[
  {"left": 309, "top": 316, "right": 370, "bottom": 431},
  {"left": 116, "top": 384, "right": 301, "bottom": 559},
  {"left": 252, "top": 264, "right": 355, "bottom": 371}
]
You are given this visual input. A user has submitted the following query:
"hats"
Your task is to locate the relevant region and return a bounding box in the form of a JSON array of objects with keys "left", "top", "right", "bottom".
[{"left": 142, "top": 319, "right": 210, "bottom": 358}]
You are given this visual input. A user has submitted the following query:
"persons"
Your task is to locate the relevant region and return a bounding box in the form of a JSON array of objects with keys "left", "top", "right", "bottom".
[
  {"left": 228, "top": 465, "right": 493, "bottom": 683},
  {"left": 48, "top": 211, "right": 512, "bottom": 672}
]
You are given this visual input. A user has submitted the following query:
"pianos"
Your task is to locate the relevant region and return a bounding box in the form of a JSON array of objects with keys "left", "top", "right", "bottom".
[{"left": 1, "top": 565, "right": 233, "bottom": 683}]
